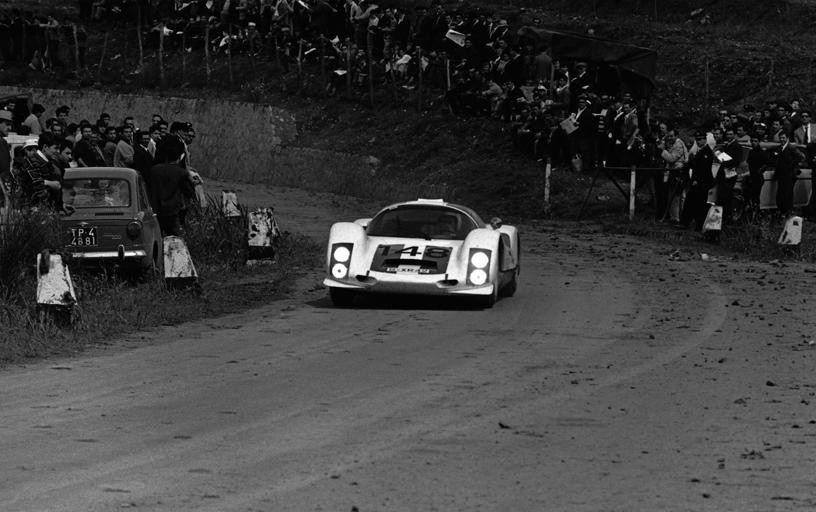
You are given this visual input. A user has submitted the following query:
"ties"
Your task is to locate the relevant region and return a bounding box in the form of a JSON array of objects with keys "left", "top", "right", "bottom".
[{"left": 804, "top": 127, "right": 808, "bottom": 145}]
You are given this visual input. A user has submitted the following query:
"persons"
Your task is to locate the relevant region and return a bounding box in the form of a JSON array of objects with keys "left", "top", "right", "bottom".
[{"left": 1, "top": 0, "right": 814, "bottom": 254}]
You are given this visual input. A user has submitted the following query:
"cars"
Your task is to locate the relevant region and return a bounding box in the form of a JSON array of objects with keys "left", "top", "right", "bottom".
[
  {"left": 53, "top": 164, "right": 166, "bottom": 285},
  {"left": 667, "top": 141, "right": 816, "bottom": 225}
]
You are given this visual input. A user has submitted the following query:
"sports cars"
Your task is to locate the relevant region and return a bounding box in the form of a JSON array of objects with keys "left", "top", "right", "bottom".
[{"left": 317, "top": 195, "right": 526, "bottom": 311}]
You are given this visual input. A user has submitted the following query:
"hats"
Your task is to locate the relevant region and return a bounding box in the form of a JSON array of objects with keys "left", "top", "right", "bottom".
[{"left": 693, "top": 130, "right": 706, "bottom": 139}]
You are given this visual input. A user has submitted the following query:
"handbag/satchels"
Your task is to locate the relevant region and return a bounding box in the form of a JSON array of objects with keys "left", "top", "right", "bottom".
[{"left": 672, "top": 164, "right": 691, "bottom": 196}]
